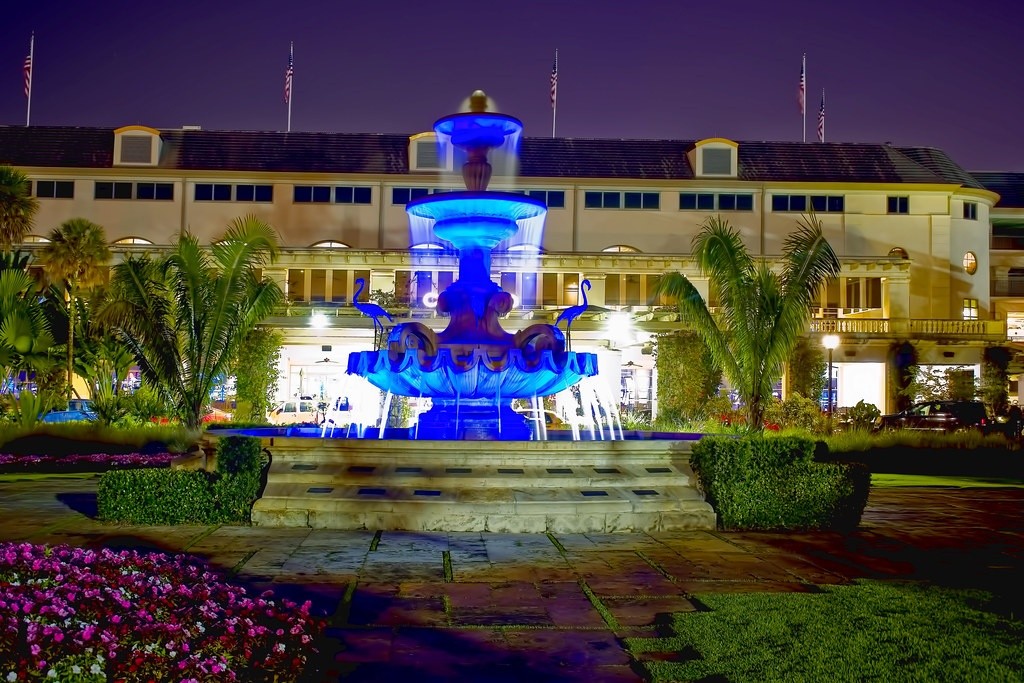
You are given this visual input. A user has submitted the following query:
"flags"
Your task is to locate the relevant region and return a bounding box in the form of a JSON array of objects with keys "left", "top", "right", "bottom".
[
  {"left": 282, "top": 51, "right": 293, "bottom": 104},
  {"left": 817, "top": 91, "right": 824, "bottom": 141},
  {"left": 549, "top": 57, "right": 557, "bottom": 108},
  {"left": 798, "top": 59, "right": 804, "bottom": 114},
  {"left": 24, "top": 41, "right": 32, "bottom": 97}
]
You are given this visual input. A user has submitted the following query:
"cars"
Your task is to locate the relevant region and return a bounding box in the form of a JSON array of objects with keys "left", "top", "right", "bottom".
[
  {"left": 269, "top": 397, "right": 361, "bottom": 428},
  {"left": 874, "top": 400, "right": 1024, "bottom": 436}
]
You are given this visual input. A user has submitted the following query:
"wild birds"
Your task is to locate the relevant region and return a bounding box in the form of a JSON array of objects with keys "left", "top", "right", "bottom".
[
  {"left": 555, "top": 279, "right": 591, "bottom": 351},
  {"left": 352, "top": 277, "right": 394, "bottom": 351}
]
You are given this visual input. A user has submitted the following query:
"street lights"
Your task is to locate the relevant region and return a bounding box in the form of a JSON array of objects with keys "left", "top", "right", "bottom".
[{"left": 822, "top": 331, "right": 841, "bottom": 421}]
